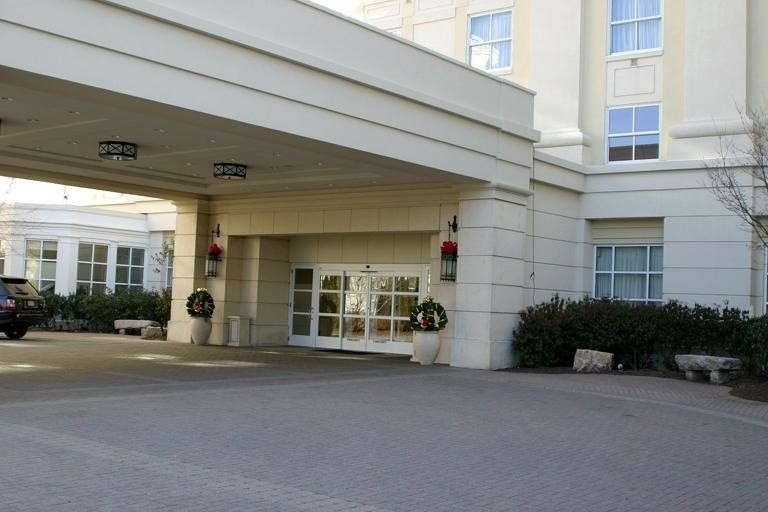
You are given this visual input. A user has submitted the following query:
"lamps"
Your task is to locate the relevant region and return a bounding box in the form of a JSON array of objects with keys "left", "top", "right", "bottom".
[
  {"left": 440, "top": 215, "right": 457, "bottom": 282},
  {"left": 213, "top": 162, "right": 247, "bottom": 179},
  {"left": 98, "top": 142, "right": 138, "bottom": 161},
  {"left": 205, "top": 224, "right": 222, "bottom": 277}
]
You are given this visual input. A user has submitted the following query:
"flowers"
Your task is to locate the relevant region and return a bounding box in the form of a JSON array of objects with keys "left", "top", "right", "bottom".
[
  {"left": 186, "top": 287, "right": 215, "bottom": 318},
  {"left": 409, "top": 296, "right": 448, "bottom": 332}
]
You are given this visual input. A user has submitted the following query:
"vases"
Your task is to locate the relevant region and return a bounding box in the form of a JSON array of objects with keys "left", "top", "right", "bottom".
[
  {"left": 411, "top": 331, "right": 440, "bottom": 366},
  {"left": 189, "top": 316, "right": 212, "bottom": 344}
]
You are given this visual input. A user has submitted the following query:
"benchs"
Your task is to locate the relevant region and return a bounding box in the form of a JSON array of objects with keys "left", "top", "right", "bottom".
[
  {"left": 113, "top": 319, "right": 159, "bottom": 334},
  {"left": 675, "top": 355, "right": 742, "bottom": 384}
]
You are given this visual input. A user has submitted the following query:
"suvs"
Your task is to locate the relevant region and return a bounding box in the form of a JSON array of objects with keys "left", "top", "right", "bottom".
[{"left": 0, "top": 275, "right": 50, "bottom": 340}]
